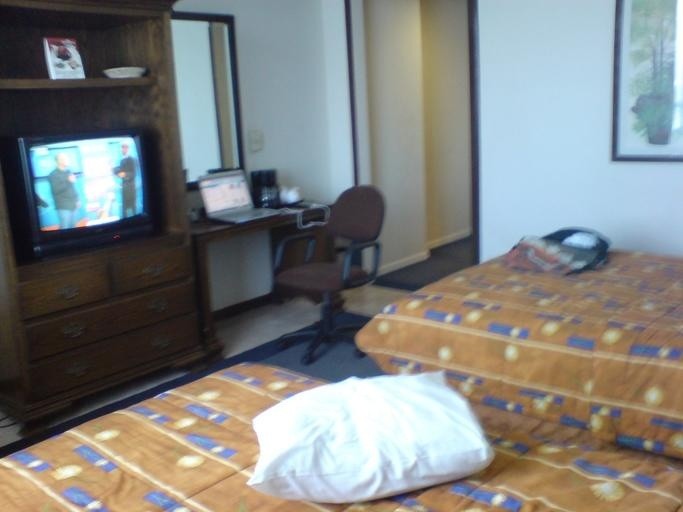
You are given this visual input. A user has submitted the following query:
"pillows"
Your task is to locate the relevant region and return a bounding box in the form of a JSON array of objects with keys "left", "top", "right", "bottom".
[{"left": 246, "top": 368, "right": 496, "bottom": 504}]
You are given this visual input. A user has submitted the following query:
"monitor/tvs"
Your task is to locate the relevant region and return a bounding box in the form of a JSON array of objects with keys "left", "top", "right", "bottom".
[{"left": 6, "top": 125, "right": 159, "bottom": 259}]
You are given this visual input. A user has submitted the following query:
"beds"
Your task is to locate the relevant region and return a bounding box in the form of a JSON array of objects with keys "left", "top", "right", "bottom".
[
  {"left": 1, "top": 361, "right": 683, "bottom": 511},
  {"left": 377, "top": 229, "right": 678, "bottom": 460},
  {"left": 272, "top": 184, "right": 387, "bottom": 355}
]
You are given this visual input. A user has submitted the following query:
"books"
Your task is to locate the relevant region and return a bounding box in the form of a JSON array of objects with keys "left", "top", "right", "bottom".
[{"left": 43, "top": 38, "right": 85, "bottom": 80}]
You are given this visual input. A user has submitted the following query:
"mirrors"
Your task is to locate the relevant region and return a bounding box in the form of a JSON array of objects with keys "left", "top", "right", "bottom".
[{"left": 168, "top": 11, "right": 245, "bottom": 192}]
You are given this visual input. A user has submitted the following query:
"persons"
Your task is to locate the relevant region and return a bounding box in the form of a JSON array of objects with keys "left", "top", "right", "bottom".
[
  {"left": 48, "top": 153, "right": 81, "bottom": 230},
  {"left": 110, "top": 142, "right": 140, "bottom": 217}
]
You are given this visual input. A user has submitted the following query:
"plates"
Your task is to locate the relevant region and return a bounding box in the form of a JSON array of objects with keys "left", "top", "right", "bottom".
[{"left": 103, "top": 66, "right": 147, "bottom": 79}]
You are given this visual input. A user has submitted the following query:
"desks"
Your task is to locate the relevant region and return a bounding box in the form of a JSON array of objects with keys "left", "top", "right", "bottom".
[{"left": 191, "top": 197, "right": 352, "bottom": 372}]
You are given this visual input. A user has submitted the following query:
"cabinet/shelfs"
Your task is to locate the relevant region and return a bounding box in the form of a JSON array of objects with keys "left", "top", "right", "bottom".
[{"left": 2, "top": 4, "right": 224, "bottom": 438}]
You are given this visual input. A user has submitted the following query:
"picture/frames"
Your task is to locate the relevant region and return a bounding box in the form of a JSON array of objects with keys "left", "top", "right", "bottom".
[{"left": 612, "top": 0, "right": 683, "bottom": 162}]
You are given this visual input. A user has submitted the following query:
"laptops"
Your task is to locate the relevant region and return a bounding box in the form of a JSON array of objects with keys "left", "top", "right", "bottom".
[{"left": 195, "top": 170, "right": 283, "bottom": 222}]
